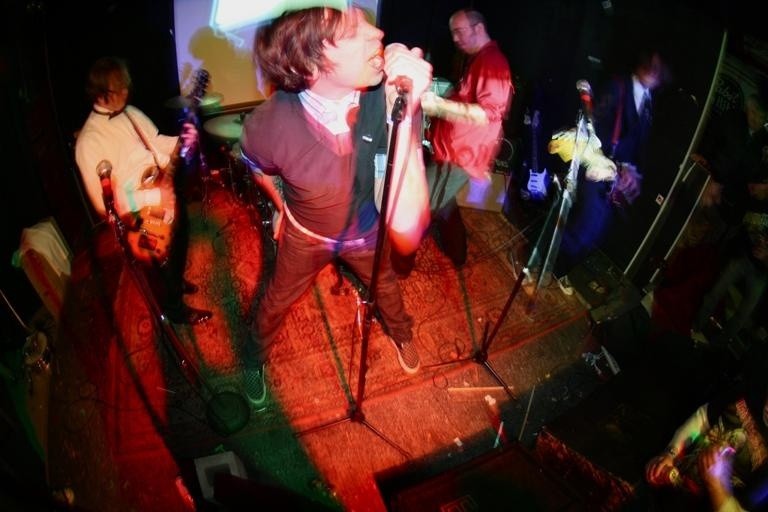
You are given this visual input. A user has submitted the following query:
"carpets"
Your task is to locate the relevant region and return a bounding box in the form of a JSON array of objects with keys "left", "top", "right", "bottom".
[{"left": 104, "top": 198, "right": 592, "bottom": 465}]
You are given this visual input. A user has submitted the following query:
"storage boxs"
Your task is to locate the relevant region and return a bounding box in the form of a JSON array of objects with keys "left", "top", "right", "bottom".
[{"left": 457, "top": 173, "right": 513, "bottom": 213}]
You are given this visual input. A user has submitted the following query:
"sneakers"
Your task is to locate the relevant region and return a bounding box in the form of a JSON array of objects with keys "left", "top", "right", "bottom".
[
  {"left": 557, "top": 274, "right": 574, "bottom": 297},
  {"left": 241, "top": 364, "right": 266, "bottom": 404},
  {"left": 389, "top": 335, "right": 420, "bottom": 374}
]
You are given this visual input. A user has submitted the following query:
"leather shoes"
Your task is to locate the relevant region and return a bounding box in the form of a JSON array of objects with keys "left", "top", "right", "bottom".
[
  {"left": 182, "top": 279, "right": 198, "bottom": 294},
  {"left": 187, "top": 308, "right": 212, "bottom": 324}
]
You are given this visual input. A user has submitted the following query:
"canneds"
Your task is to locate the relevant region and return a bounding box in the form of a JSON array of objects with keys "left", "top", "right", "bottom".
[{"left": 648, "top": 465, "right": 678, "bottom": 488}]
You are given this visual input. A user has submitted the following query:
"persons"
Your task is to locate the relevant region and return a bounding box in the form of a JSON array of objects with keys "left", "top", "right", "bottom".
[
  {"left": 235, "top": 7, "right": 422, "bottom": 403},
  {"left": 544, "top": 50, "right": 668, "bottom": 295},
  {"left": 391, "top": 8, "right": 513, "bottom": 279},
  {"left": 74, "top": 55, "right": 213, "bottom": 324},
  {"left": 631, "top": 96, "right": 767, "bottom": 512}
]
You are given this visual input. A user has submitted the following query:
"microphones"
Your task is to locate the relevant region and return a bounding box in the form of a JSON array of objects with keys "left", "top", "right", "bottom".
[
  {"left": 97, "top": 160, "right": 117, "bottom": 223},
  {"left": 383, "top": 42, "right": 411, "bottom": 95},
  {"left": 576, "top": 78, "right": 595, "bottom": 123}
]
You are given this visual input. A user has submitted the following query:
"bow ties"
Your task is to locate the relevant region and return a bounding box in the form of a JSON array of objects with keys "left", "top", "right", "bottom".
[{"left": 108, "top": 108, "right": 126, "bottom": 120}]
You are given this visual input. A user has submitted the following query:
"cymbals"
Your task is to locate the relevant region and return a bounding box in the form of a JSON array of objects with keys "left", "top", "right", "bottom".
[
  {"left": 166, "top": 93, "right": 224, "bottom": 108},
  {"left": 204, "top": 113, "right": 251, "bottom": 138}
]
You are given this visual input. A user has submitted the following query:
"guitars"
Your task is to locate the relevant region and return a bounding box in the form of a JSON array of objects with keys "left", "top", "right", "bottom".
[{"left": 127, "top": 70, "right": 209, "bottom": 267}]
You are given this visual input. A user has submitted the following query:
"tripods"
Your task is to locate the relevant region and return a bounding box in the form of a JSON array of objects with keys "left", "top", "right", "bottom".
[
  {"left": 293, "top": 121, "right": 415, "bottom": 461},
  {"left": 420, "top": 189, "right": 562, "bottom": 399},
  {"left": 453, "top": 119, "right": 588, "bottom": 315}
]
